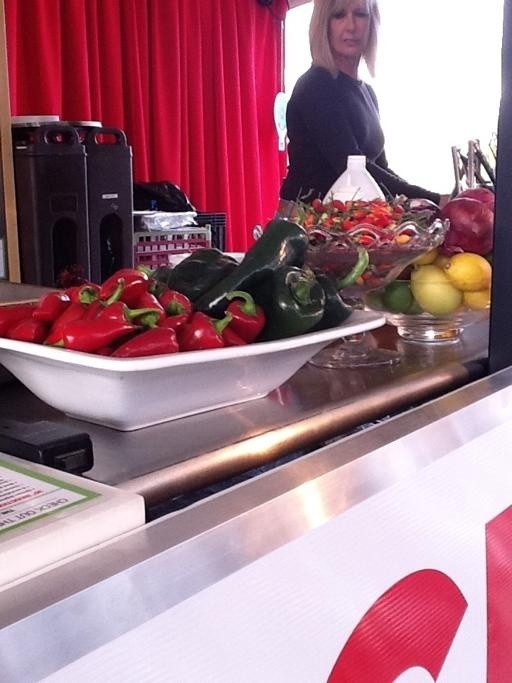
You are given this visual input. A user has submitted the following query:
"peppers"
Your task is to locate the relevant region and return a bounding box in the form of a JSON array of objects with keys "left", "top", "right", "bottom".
[{"left": 1, "top": 215, "right": 371, "bottom": 358}]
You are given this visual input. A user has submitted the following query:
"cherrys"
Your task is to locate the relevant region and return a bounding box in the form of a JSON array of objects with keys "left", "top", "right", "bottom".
[{"left": 283, "top": 189, "right": 414, "bottom": 288}]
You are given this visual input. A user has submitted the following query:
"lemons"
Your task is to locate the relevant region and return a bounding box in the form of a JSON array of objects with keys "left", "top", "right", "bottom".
[{"left": 384, "top": 250, "right": 493, "bottom": 318}]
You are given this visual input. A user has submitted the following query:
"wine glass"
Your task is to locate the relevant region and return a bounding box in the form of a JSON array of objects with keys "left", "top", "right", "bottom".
[{"left": 273, "top": 196, "right": 452, "bottom": 373}]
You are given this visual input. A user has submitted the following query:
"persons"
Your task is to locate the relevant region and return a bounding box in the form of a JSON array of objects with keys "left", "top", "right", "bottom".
[{"left": 276, "top": 0, "right": 451, "bottom": 221}]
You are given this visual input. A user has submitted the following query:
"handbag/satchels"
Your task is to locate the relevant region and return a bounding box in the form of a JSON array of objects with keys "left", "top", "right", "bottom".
[{"left": 134, "top": 181, "right": 193, "bottom": 212}]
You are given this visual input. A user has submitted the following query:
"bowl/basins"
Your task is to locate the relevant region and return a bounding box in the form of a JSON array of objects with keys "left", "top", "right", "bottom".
[
  {"left": 353, "top": 273, "right": 493, "bottom": 347},
  {"left": 2, "top": 305, "right": 387, "bottom": 433}
]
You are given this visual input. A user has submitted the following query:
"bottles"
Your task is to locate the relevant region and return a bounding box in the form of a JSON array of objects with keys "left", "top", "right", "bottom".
[{"left": 322, "top": 153, "right": 389, "bottom": 203}]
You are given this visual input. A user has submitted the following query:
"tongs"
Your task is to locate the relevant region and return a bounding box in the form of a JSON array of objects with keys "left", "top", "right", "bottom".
[{"left": 449, "top": 138, "right": 496, "bottom": 195}]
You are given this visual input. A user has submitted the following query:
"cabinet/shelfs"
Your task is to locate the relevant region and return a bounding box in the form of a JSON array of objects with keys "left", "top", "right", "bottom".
[{"left": 132, "top": 212, "right": 227, "bottom": 257}]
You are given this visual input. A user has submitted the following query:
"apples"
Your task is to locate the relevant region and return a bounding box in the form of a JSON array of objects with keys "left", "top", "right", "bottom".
[{"left": 435, "top": 187, "right": 493, "bottom": 260}]
